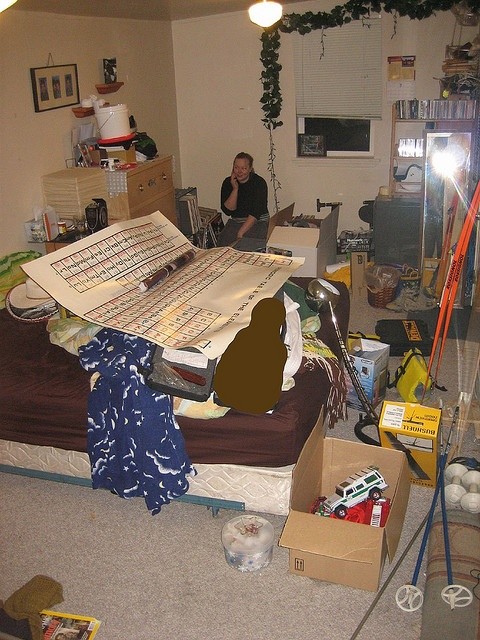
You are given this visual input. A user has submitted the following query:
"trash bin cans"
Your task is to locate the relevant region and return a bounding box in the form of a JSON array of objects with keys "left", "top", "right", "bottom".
[{"left": 366, "top": 265, "right": 401, "bottom": 309}]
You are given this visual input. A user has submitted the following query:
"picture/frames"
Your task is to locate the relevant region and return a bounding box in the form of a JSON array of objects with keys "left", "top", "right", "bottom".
[
  {"left": 298, "top": 133, "right": 327, "bottom": 157},
  {"left": 31, "top": 63, "right": 80, "bottom": 113}
]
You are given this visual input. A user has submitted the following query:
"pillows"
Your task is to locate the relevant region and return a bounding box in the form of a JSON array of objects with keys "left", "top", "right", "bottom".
[{"left": 1, "top": 249, "right": 41, "bottom": 309}]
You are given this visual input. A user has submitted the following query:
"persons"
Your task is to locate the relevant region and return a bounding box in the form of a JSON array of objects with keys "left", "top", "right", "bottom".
[{"left": 214, "top": 152, "right": 270, "bottom": 252}]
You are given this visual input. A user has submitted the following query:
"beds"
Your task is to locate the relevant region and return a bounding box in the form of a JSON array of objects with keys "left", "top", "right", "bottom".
[{"left": 1, "top": 253, "right": 350, "bottom": 517}]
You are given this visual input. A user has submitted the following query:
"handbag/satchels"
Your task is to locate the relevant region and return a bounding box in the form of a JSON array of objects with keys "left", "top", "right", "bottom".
[
  {"left": 395, "top": 267, "right": 420, "bottom": 299},
  {"left": 375, "top": 319, "right": 432, "bottom": 357},
  {"left": 387, "top": 347, "right": 447, "bottom": 405}
]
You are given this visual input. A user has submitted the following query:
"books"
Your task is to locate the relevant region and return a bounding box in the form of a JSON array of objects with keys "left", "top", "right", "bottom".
[
  {"left": 397, "top": 138, "right": 424, "bottom": 158},
  {"left": 395, "top": 98, "right": 478, "bottom": 121},
  {"left": 42, "top": 209, "right": 60, "bottom": 242}
]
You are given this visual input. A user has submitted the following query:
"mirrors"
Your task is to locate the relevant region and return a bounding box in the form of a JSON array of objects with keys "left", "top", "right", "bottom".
[{"left": 419, "top": 127, "right": 474, "bottom": 309}]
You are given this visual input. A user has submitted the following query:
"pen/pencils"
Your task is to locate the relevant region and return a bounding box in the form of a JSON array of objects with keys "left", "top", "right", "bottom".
[{"left": 76, "top": 143, "right": 94, "bottom": 167}]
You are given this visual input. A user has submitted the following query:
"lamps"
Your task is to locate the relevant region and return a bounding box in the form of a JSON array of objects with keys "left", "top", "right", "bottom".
[{"left": 247, "top": 1, "right": 284, "bottom": 29}]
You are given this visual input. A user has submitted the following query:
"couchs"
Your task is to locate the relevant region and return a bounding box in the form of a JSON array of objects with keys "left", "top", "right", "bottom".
[{"left": 422, "top": 508, "right": 480, "bottom": 640}]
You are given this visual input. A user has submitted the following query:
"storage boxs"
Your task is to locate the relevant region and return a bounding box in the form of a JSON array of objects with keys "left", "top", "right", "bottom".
[
  {"left": 279, "top": 405, "right": 412, "bottom": 593},
  {"left": 377, "top": 400, "right": 443, "bottom": 489},
  {"left": 346, "top": 337, "right": 390, "bottom": 415},
  {"left": 264, "top": 202, "right": 339, "bottom": 277}
]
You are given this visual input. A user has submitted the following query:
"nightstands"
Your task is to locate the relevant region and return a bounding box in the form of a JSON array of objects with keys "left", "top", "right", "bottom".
[{"left": 29, "top": 227, "right": 94, "bottom": 253}]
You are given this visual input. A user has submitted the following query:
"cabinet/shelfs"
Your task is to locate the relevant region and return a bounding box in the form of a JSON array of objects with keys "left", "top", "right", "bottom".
[
  {"left": 390, "top": 98, "right": 479, "bottom": 199},
  {"left": 107, "top": 155, "right": 179, "bottom": 231}
]
